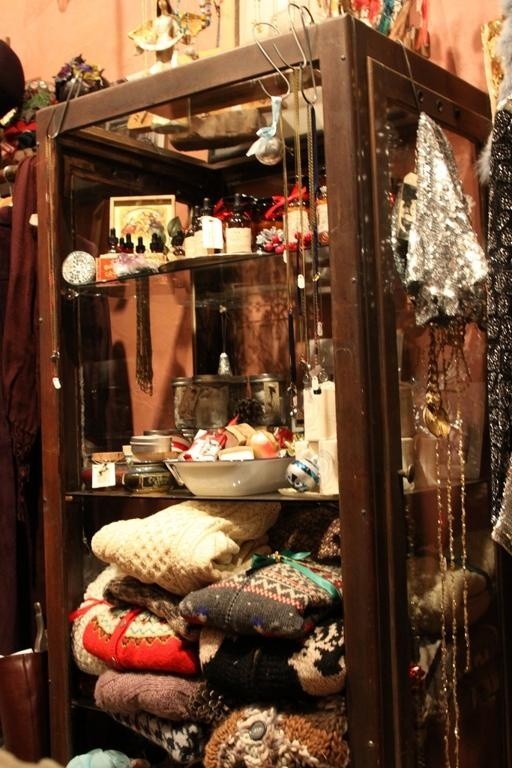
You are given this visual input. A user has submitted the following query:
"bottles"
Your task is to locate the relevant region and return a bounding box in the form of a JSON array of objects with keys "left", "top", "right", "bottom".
[
  {"left": 174, "top": 167, "right": 330, "bottom": 264},
  {"left": 106, "top": 225, "right": 164, "bottom": 253}
]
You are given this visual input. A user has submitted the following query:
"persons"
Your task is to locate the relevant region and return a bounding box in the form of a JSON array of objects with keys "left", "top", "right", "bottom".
[{"left": 135, "top": 1, "right": 180, "bottom": 73}]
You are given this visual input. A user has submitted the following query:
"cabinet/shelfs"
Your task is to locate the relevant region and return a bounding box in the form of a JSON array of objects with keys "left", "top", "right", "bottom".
[{"left": 31, "top": 11, "right": 511, "bottom": 768}]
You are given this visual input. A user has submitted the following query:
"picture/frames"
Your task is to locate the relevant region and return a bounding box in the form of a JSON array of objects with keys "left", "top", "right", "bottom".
[{"left": 108, "top": 193, "right": 176, "bottom": 246}]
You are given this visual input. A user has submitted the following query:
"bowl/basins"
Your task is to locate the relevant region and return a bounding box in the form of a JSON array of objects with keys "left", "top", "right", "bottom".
[
  {"left": 170, "top": 376, "right": 251, "bottom": 429},
  {"left": 165, "top": 456, "right": 294, "bottom": 499}
]
